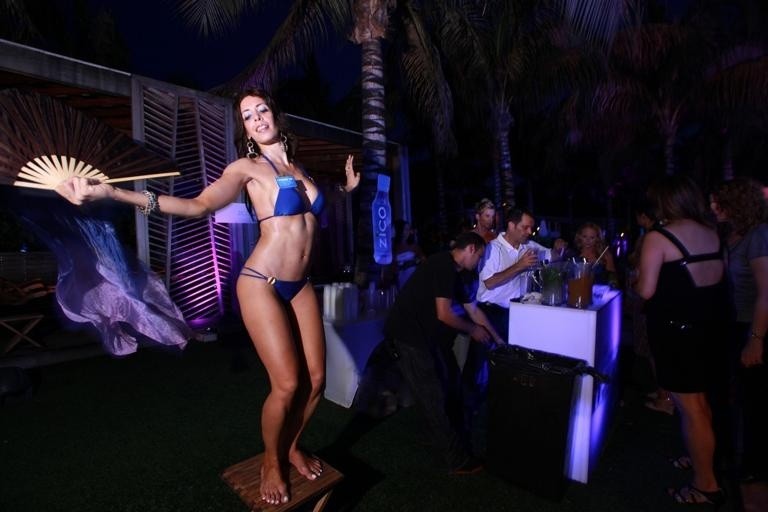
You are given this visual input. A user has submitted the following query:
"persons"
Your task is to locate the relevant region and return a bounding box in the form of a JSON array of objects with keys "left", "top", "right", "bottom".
[{"left": 66, "top": 85, "right": 365, "bottom": 505}]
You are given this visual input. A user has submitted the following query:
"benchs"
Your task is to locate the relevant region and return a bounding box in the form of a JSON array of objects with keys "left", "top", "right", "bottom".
[{"left": 323, "top": 314, "right": 409, "bottom": 408}]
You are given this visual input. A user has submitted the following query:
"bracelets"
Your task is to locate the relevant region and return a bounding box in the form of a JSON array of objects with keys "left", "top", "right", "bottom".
[
  {"left": 333, "top": 182, "right": 347, "bottom": 200},
  {"left": 138, "top": 190, "right": 159, "bottom": 219}
]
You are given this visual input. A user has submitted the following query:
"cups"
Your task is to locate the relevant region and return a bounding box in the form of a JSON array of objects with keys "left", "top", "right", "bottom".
[
  {"left": 566, "top": 262, "right": 593, "bottom": 308},
  {"left": 531, "top": 264, "right": 564, "bottom": 306}
]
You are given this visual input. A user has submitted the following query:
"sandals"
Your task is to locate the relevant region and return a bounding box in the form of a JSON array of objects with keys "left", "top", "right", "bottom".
[
  {"left": 672, "top": 453, "right": 692, "bottom": 470},
  {"left": 665, "top": 478, "right": 729, "bottom": 505}
]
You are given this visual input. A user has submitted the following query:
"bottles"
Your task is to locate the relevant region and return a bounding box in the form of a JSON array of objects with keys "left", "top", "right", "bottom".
[{"left": 370, "top": 172, "right": 394, "bottom": 266}]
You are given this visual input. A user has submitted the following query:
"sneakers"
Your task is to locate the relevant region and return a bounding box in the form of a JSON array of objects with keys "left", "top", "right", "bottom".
[
  {"left": 642, "top": 390, "right": 676, "bottom": 415},
  {"left": 447, "top": 459, "right": 490, "bottom": 476}
]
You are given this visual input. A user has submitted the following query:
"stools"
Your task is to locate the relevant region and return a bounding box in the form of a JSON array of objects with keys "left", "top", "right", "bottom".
[{"left": 222, "top": 446, "right": 345, "bottom": 511}]
